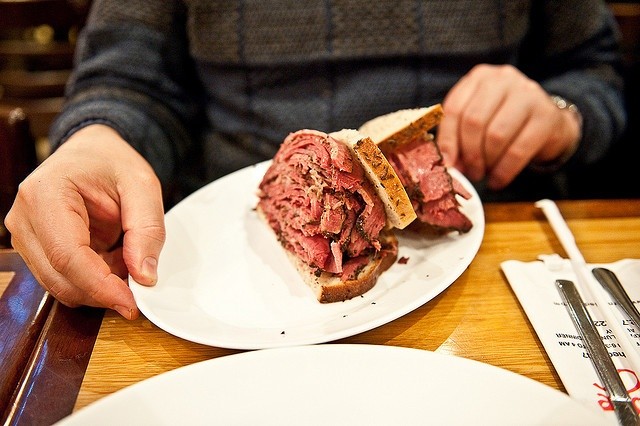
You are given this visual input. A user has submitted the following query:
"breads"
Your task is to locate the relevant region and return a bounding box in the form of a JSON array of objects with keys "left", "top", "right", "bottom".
[
  {"left": 251, "top": 129, "right": 419, "bottom": 305},
  {"left": 359, "top": 103, "right": 473, "bottom": 235}
]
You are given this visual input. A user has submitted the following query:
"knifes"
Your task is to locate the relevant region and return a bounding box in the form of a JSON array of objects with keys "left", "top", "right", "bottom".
[{"left": 556, "top": 279, "right": 635, "bottom": 425}]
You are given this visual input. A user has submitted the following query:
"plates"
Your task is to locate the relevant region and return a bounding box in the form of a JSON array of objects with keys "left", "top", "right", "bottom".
[
  {"left": 45, "top": 347, "right": 623, "bottom": 426},
  {"left": 129, "top": 151, "right": 487, "bottom": 349}
]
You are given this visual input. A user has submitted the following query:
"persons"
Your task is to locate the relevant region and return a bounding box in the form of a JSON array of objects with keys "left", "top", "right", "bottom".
[{"left": 3, "top": 1, "right": 640, "bottom": 320}]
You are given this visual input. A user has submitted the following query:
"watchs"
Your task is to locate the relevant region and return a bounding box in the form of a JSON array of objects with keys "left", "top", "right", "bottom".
[{"left": 551, "top": 94, "right": 583, "bottom": 138}]
